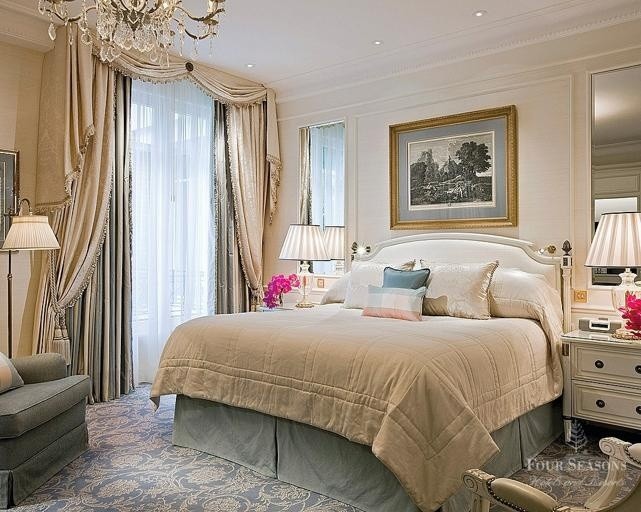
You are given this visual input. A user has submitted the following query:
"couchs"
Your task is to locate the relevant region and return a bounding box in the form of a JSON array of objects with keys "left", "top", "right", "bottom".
[{"left": 0, "top": 350, "right": 92, "bottom": 509}]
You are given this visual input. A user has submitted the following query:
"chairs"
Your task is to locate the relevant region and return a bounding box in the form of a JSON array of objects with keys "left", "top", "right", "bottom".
[{"left": 462, "top": 437, "right": 640, "bottom": 511}]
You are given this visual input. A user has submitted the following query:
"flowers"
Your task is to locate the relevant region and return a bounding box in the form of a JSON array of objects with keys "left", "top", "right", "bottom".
[
  {"left": 262, "top": 274, "right": 300, "bottom": 309},
  {"left": 618, "top": 294, "right": 641, "bottom": 338}
]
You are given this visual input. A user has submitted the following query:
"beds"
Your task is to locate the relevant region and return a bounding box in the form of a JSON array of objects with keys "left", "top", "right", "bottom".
[{"left": 148, "top": 230, "right": 574, "bottom": 512}]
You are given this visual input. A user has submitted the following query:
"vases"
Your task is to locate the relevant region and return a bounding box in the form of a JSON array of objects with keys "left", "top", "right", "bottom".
[{"left": 281, "top": 290, "right": 301, "bottom": 308}]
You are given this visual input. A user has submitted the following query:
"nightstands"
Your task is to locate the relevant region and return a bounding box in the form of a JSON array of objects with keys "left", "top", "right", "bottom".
[{"left": 560, "top": 320, "right": 641, "bottom": 441}]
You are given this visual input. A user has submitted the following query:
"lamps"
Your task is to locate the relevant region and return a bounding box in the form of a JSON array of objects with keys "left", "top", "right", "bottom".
[
  {"left": 278, "top": 225, "right": 330, "bottom": 309},
  {"left": 1, "top": 197, "right": 62, "bottom": 360},
  {"left": 36, "top": 0, "right": 226, "bottom": 70},
  {"left": 323, "top": 226, "right": 346, "bottom": 278},
  {"left": 584, "top": 211, "right": 641, "bottom": 342}
]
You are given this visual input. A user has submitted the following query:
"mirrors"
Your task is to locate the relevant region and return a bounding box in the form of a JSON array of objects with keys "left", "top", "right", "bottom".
[
  {"left": 298, "top": 118, "right": 347, "bottom": 279},
  {"left": 585, "top": 62, "right": 641, "bottom": 287}
]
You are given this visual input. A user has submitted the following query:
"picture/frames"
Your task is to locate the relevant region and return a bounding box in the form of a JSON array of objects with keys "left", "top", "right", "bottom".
[
  {"left": 388, "top": 103, "right": 520, "bottom": 229},
  {"left": 0, "top": 149, "right": 21, "bottom": 255}
]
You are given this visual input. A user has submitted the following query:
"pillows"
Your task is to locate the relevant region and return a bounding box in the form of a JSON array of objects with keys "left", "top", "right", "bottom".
[
  {"left": 361, "top": 283, "right": 428, "bottom": 322},
  {"left": 343, "top": 260, "right": 416, "bottom": 310},
  {"left": 420, "top": 258, "right": 499, "bottom": 320},
  {"left": 382, "top": 266, "right": 431, "bottom": 289}
]
